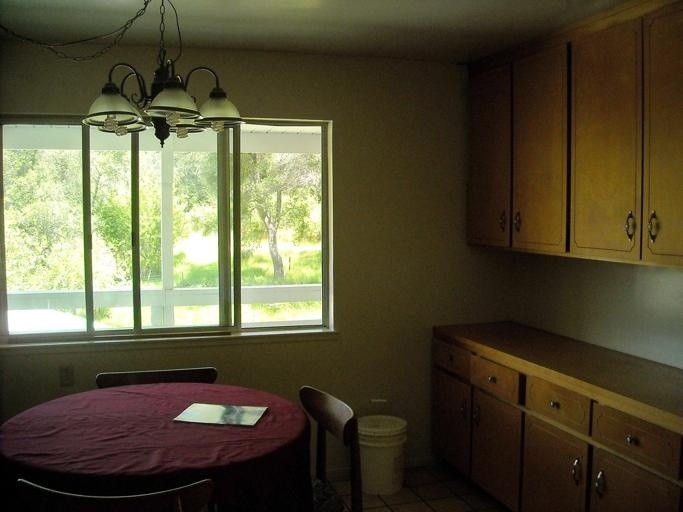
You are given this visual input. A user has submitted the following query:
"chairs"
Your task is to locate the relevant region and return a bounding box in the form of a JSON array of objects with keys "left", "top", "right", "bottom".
[
  {"left": 96, "top": 367, "right": 218, "bottom": 388},
  {"left": 14, "top": 476, "right": 214, "bottom": 511},
  {"left": 299, "top": 385, "right": 363, "bottom": 511}
]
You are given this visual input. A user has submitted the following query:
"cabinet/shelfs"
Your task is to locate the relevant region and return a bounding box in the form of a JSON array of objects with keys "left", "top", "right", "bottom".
[
  {"left": 567, "top": 0, "right": 683, "bottom": 267},
  {"left": 431, "top": 334, "right": 523, "bottom": 512},
  {"left": 520, "top": 376, "right": 683, "bottom": 511},
  {"left": 467, "top": 43, "right": 569, "bottom": 256}
]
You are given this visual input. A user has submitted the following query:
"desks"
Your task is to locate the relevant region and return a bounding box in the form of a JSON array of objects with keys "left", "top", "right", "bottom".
[{"left": 0, "top": 381, "right": 312, "bottom": 512}]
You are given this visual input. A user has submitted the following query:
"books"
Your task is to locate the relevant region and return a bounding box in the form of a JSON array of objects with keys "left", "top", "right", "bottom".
[{"left": 173, "top": 402, "right": 268, "bottom": 427}]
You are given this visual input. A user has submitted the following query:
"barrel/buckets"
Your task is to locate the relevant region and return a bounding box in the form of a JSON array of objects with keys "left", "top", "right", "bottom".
[{"left": 356, "top": 399, "right": 409, "bottom": 496}]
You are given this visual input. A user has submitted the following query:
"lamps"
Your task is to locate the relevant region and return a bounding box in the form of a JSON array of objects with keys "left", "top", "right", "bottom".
[{"left": 81, "top": 0, "right": 248, "bottom": 149}]
